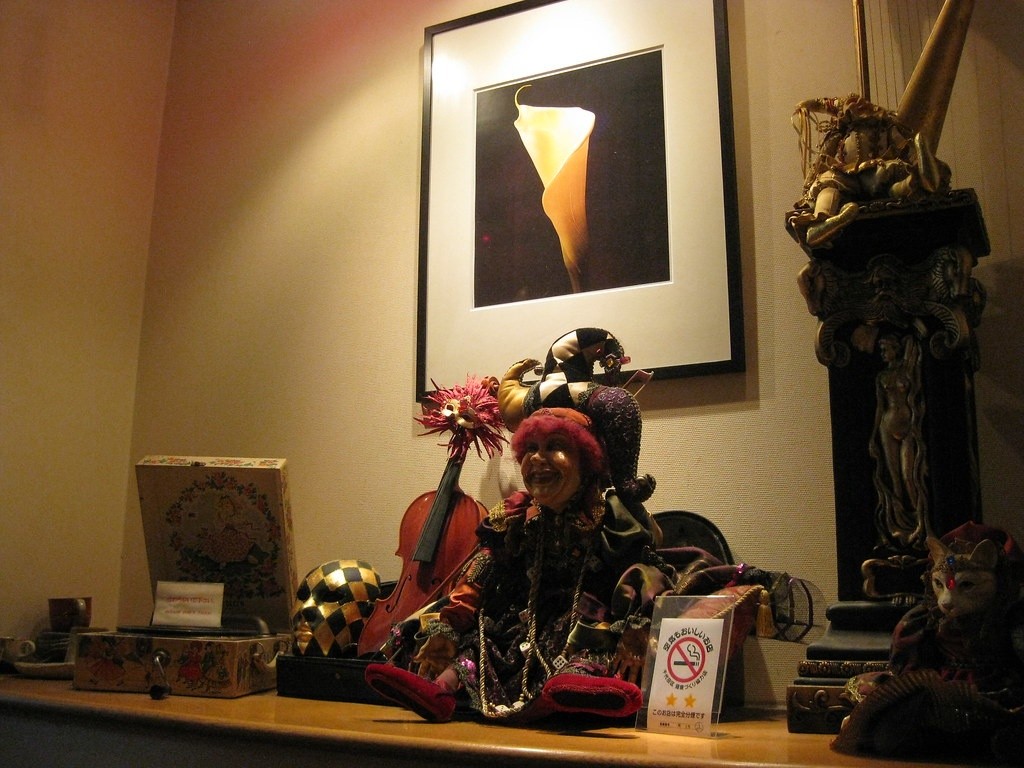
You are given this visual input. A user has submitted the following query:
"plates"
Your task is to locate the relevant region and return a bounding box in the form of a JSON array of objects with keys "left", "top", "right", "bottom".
[{"left": 16, "top": 662, "right": 74, "bottom": 678}]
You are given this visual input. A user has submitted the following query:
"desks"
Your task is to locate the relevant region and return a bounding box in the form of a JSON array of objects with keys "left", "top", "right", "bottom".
[{"left": 0, "top": 676, "right": 961, "bottom": 768}]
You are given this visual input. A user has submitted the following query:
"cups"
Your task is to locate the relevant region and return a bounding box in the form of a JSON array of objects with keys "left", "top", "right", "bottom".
[
  {"left": 0, "top": 637, "right": 35, "bottom": 664},
  {"left": 49, "top": 596, "right": 91, "bottom": 633}
]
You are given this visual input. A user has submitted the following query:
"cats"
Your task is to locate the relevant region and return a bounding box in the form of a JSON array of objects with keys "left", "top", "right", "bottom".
[{"left": 925, "top": 536, "right": 998, "bottom": 618}]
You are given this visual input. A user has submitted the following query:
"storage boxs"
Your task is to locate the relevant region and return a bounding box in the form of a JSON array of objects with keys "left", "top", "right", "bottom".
[{"left": 76, "top": 453, "right": 299, "bottom": 699}]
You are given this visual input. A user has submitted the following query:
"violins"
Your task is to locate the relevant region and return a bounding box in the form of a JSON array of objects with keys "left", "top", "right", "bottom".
[{"left": 356, "top": 375, "right": 499, "bottom": 657}]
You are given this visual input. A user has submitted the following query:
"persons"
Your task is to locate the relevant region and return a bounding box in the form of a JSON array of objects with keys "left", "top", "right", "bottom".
[
  {"left": 288, "top": 560, "right": 381, "bottom": 657},
  {"left": 868, "top": 333, "right": 937, "bottom": 552},
  {"left": 370, "top": 327, "right": 684, "bottom": 724},
  {"left": 794, "top": 95, "right": 941, "bottom": 246}
]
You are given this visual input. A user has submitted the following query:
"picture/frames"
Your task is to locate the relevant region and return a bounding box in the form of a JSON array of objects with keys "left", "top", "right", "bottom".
[{"left": 414, "top": 0, "right": 746, "bottom": 404}]
